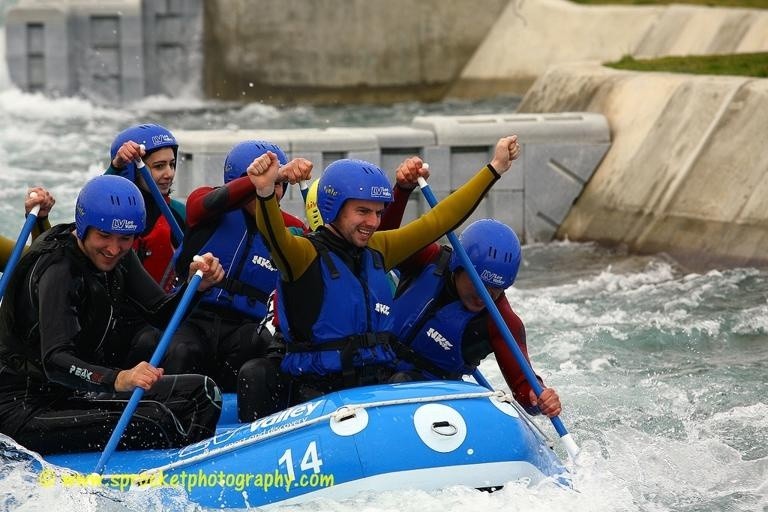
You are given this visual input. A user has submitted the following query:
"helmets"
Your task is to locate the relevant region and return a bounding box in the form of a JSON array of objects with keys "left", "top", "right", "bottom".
[{"left": 75, "top": 124, "right": 522, "bottom": 288}]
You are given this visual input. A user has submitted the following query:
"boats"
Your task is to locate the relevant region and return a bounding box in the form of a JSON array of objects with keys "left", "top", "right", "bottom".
[{"left": 0, "top": 380, "right": 569, "bottom": 509}]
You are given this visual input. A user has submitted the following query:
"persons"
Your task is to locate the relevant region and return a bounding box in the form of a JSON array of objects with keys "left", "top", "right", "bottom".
[
  {"left": 105, "top": 122, "right": 191, "bottom": 296},
  {"left": 0, "top": 185, "right": 60, "bottom": 276},
  {"left": 0, "top": 173, "right": 226, "bottom": 453},
  {"left": 236, "top": 176, "right": 323, "bottom": 423},
  {"left": 160, "top": 138, "right": 312, "bottom": 393},
  {"left": 380, "top": 152, "right": 561, "bottom": 420},
  {"left": 246, "top": 134, "right": 523, "bottom": 408}
]
